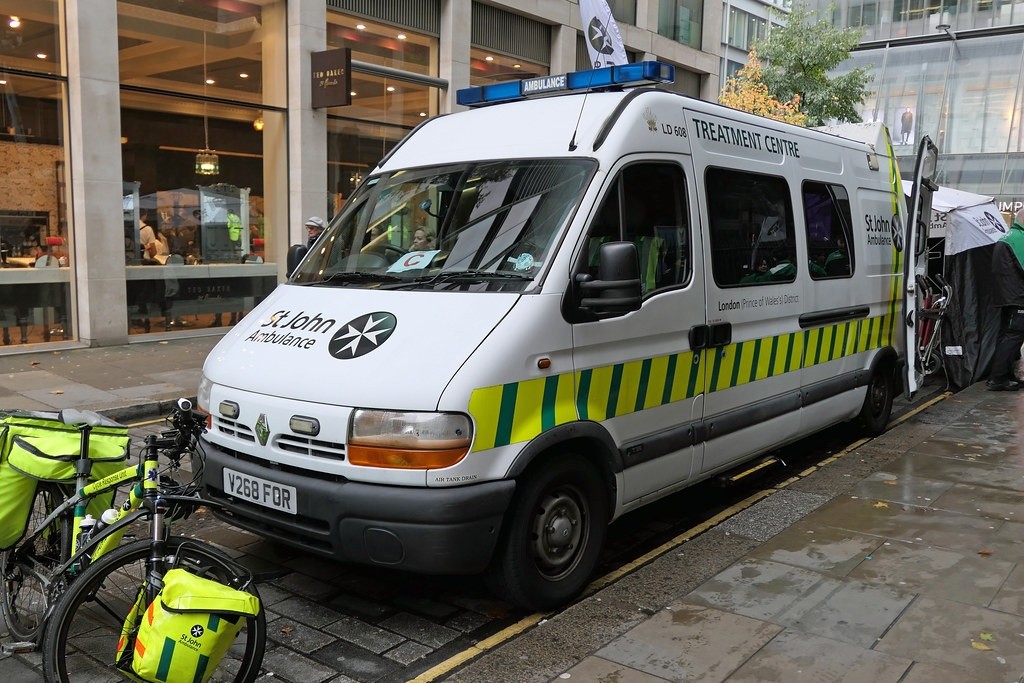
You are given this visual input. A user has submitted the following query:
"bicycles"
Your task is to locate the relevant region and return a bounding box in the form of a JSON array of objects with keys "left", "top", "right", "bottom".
[{"left": 0, "top": 396, "right": 267, "bottom": 683}]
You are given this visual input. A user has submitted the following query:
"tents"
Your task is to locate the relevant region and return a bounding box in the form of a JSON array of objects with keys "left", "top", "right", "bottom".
[{"left": 899, "top": 178, "right": 1010, "bottom": 392}]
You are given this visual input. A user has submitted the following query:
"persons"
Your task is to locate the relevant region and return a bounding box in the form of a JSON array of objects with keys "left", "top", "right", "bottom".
[
  {"left": 305, "top": 216, "right": 328, "bottom": 250},
  {"left": 414, "top": 225, "right": 437, "bottom": 251},
  {"left": 900, "top": 108, "right": 913, "bottom": 145},
  {"left": 985, "top": 207, "right": 1024, "bottom": 393},
  {"left": 139, "top": 209, "right": 157, "bottom": 259},
  {"left": 226, "top": 208, "right": 242, "bottom": 242}
]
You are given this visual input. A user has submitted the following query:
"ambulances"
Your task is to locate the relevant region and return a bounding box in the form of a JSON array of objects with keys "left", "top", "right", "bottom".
[{"left": 189, "top": 60, "right": 939, "bottom": 618}]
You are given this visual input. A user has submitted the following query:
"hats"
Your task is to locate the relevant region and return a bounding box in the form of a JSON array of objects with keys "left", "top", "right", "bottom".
[{"left": 304, "top": 216, "right": 326, "bottom": 229}]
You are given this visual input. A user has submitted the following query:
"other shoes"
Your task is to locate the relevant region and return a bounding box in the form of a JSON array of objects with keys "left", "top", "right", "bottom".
[
  {"left": 1008, "top": 373, "right": 1024, "bottom": 389},
  {"left": 986, "top": 376, "right": 1019, "bottom": 391}
]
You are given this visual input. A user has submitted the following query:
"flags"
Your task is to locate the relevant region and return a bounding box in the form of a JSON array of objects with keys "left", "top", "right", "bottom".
[{"left": 579, "top": 0, "right": 630, "bottom": 70}]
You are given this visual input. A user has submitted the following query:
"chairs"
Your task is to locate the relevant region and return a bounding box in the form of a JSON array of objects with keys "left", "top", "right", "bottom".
[{"left": 615, "top": 174, "right": 682, "bottom": 288}]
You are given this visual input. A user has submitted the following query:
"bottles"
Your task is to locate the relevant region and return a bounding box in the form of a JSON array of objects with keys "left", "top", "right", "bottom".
[
  {"left": 77, "top": 515, "right": 97, "bottom": 552},
  {"left": 83, "top": 509, "right": 118, "bottom": 548}
]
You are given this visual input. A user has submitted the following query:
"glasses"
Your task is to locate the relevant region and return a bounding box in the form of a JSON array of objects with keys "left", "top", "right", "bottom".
[{"left": 305, "top": 226, "right": 317, "bottom": 230}]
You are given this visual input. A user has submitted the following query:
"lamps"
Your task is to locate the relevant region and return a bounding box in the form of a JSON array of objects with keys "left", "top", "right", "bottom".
[
  {"left": 195, "top": 20, "right": 219, "bottom": 174},
  {"left": 936, "top": 24, "right": 956, "bottom": 41}
]
